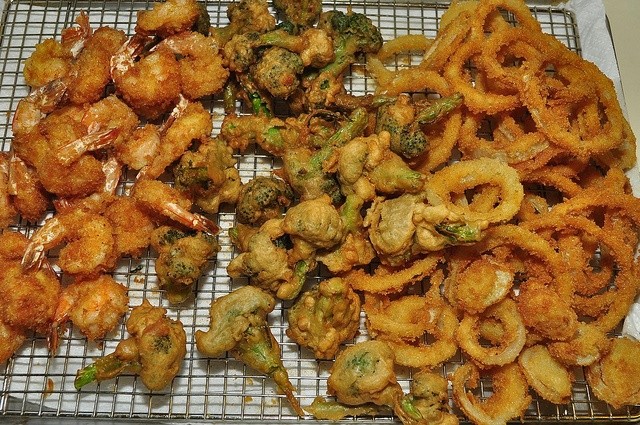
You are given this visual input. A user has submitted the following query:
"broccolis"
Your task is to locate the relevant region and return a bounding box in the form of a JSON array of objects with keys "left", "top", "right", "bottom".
[{"left": 75, "top": 0, "right": 488, "bottom": 419}]
